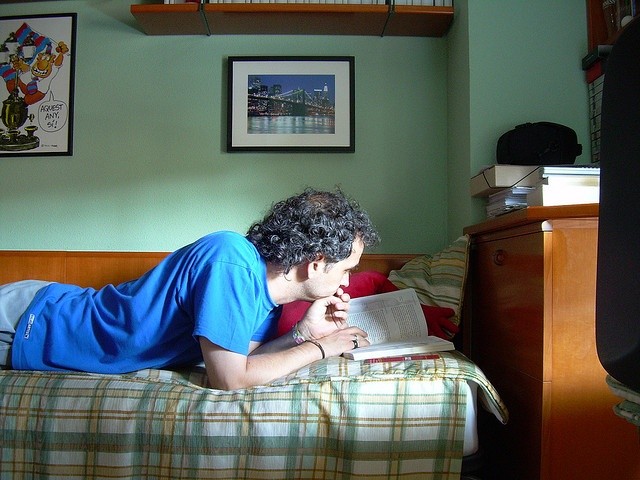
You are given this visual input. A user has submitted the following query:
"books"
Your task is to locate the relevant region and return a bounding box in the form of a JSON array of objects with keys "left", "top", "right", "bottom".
[
  {"left": 532, "top": 175, "right": 600, "bottom": 185},
  {"left": 526, "top": 184, "right": 600, "bottom": 206},
  {"left": 342, "top": 288, "right": 456, "bottom": 361}
]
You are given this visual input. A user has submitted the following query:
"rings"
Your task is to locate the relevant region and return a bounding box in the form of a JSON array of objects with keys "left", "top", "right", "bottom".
[
  {"left": 353, "top": 340, "right": 358, "bottom": 348},
  {"left": 355, "top": 335, "right": 358, "bottom": 340}
]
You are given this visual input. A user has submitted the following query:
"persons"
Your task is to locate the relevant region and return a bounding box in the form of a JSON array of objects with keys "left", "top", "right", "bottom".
[{"left": 0, "top": 182, "right": 382, "bottom": 390}]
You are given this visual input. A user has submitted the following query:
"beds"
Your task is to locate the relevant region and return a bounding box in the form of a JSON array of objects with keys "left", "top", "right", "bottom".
[{"left": 1, "top": 249, "right": 505, "bottom": 480}]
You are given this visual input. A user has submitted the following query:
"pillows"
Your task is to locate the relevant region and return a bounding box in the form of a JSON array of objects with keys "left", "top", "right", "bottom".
[
  {"left": 276, "top": 271, "right": 459, "bottom": 342},
  {"left": 388, "top": 237, "right": 472, "bottom": 342}
]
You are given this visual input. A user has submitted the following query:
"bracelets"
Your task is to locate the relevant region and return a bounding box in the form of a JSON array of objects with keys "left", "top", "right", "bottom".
[
  {"left": 292, "top": 321, "right": 305, "bottom": 345},
  {"left": 305, "top": 340, "right": 325, "bottom": 359}
]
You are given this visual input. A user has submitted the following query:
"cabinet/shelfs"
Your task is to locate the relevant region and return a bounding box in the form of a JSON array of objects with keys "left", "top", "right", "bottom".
[{"left": 465, "top": 204, "right": 640, "bottom": 480}]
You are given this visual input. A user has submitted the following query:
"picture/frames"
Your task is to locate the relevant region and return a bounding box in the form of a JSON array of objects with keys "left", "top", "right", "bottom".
[
  {"left": 0, "top": 12, "right": 77, "bottom": 156},
  {"left": 228, "top": 55, "right": 356, "bottom": 153}
]
